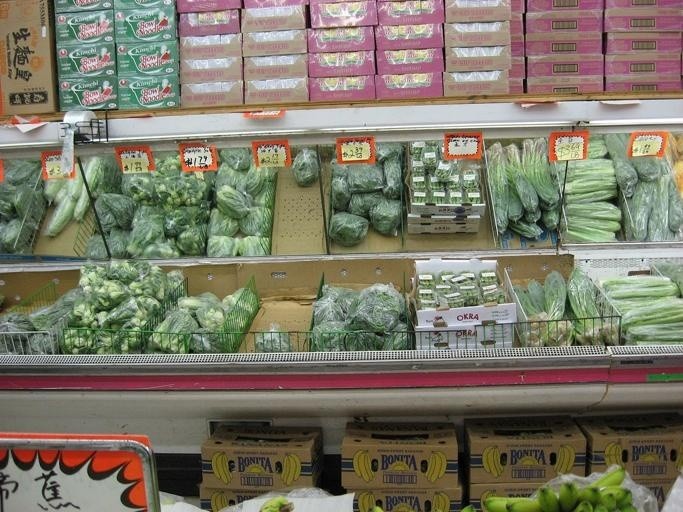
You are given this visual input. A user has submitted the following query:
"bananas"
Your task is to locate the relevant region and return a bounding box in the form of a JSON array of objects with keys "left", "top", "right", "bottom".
[
  {"left": 674, "top": 439, "right": 683, "bottom": 473},
  {"left": 553, "top": 444, "right": 576, "bottom": 474},
  {"left": 372, "top": 506, "right": 384, "bottom": 512},
  {"left": 482, "top": 445, "right": 504, "bottom": 479},
  {"left": 358, "top": 491, "right": 376, "bottom": 512},
  {"left": 259, "top": 497, "right": 294, "bottom": 512},
  {"left": 605, "top": 440, "right": 625, "bottom": 469},
  {"left": 425, "top": 451, "right": 447, "bottom": 483},
  {"left": 280, "top": 453, "right": 302, "bottom": 486},
  {"left": 353, "top": 448, "right": 375, "bottom": 482},
  {"left": 210, "top": 491, "right": 228, "bottom": 512},
  {"left": 212, "top": 452, "right": 232, "bottom": 485},
  {"left": 480, "top": 489, "right": 496, "bottom": 512},
  {"left": 483, "top": 466, "right": 639, "bottom": 512},
  {"left": 431, "top": 490, "right": 451, "bottom": 512}
]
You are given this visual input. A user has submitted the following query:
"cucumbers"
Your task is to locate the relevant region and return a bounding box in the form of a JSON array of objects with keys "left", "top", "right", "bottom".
[{"left": 43, "top": 156, "right": 100, "bottom": 235}]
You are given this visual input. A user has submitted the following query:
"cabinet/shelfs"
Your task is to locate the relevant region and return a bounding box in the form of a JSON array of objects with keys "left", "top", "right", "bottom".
[{"left": 0, "top": 93, "right": 682, "bottom": 420}]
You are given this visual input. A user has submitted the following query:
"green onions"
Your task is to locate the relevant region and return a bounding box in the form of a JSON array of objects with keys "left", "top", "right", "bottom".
[
  {"left": 486, "top": 139, "right": 560, "bottom": 238},
  {"left": 514, "top": 267, "right": 615, "bottom": 347}
]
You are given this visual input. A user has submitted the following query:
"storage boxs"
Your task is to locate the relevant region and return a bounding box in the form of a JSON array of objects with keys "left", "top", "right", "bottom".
[
  {"left": 241, "top": 3, "right": 311, "bottom": 107},
  {"left": 174, "top": 0, "right": 243, "bottom": 111},
  {"left": 347, "top": 488, "right": 463, "bottom": 512},
  {"left": 641, "top": 482, "right": 673, "bottom": 509},
  {"left": 604, "top": 1, "right": 682, "bottom": 95},
  {"left": 113, "top": 2, "right": 180, "bottom": 110},
  {"left": 339, "top": 427, "right": 461, "bottom": 487},
  {"left": 305, "top": 1, "right": 378, "bottom": 103},
  {"left": 440, "top": 0, "right": 527, "bottom": 100},
  {"left": 469, "top": 482, "right": 544, "bottom": 509},
  {"left": 461, "top": 421, "right": 589, "bottom": 480},
  {"left": 1, "top": 2, "right": 60, "bottom": 115},
  {"left": 201, "top": 428, "right": 326, "bottom": 487},
  {"left": 199, "top": 487, "right": 262, "bottom": 509},
  {"left": 526, "top": 1, "right": 606, "bottom": 94},
  {"left": 580, "top": 421, "right": 683, "bottom": 480},
  {"left": 375, "top": 1, "right": 445, "bottom": 103},
  {"left": 52, "top": 1, "right": 116, "bottom": 111}
]
